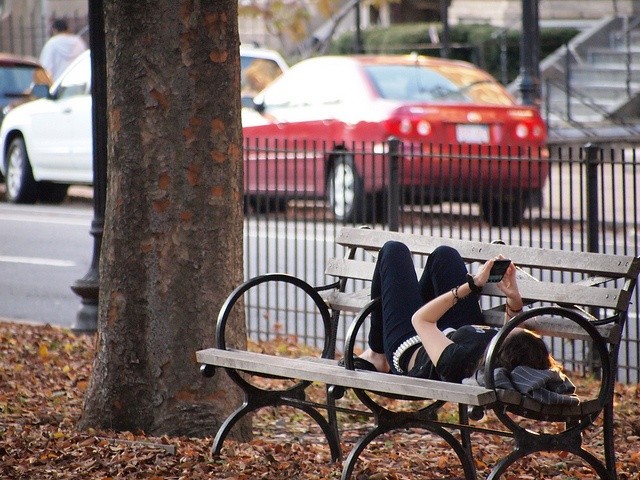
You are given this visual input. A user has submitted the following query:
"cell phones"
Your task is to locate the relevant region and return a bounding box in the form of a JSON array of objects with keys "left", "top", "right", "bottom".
[{"left": 487, "top": 259, "right": 512, "bottom": 285}]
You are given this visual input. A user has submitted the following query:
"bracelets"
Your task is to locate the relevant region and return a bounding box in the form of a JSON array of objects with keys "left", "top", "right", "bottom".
[
  {"left": 451, "top": 286, "right": 459, "bottom": 305},
  {"left": 507, "top": 311, "right": 512, "bottom": 319},
  {"left": 506, "top": 300, "right": 523, "bottom": 313}
]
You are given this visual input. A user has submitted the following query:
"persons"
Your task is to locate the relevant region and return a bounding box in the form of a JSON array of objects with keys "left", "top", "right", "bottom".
[
  {"left": 358, "top": 241, "right": 550, "bottom": 384},
  {"left": 39, "top": 20, "right": 88, "bottom": 84}
]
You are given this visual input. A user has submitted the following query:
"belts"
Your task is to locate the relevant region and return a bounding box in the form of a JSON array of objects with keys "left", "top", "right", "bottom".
[{"left": 393, "top": 327, "right": 458, "bottom": 373}]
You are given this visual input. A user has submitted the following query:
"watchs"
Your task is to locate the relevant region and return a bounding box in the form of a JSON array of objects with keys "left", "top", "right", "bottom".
[{"left": 466, "top": 274, "right": 483, "bottom": 293}]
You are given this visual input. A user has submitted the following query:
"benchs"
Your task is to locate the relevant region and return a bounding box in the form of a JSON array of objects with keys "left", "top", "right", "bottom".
[{"left": 197, "top": 226, "right": 638, "bottom": 479}]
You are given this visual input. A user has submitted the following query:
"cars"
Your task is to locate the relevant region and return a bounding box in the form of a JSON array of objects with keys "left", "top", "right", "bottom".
[
  {"left": 0, "top": 42, "right": 288, "bottom": 204},
  {"left": 240, "top": 54, "right": 549, "bottom": 227}
]
